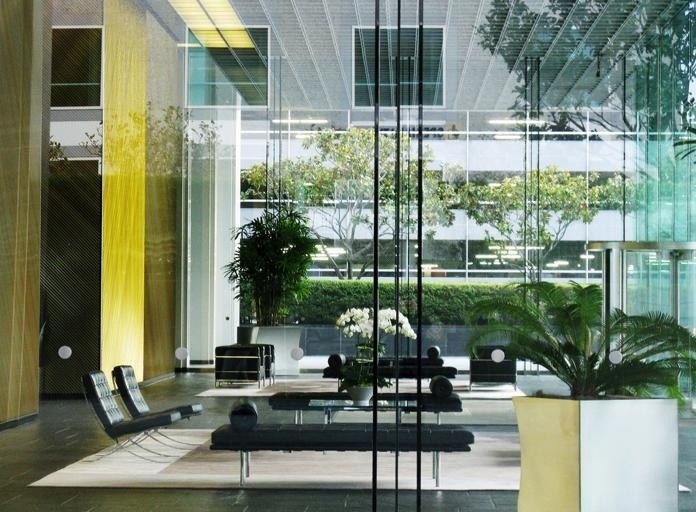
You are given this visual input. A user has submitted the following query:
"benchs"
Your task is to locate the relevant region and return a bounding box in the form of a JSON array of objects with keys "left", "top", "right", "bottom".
[
  {"left": 210, "top": 423, "right": 475, "bottom": 487},
  {"left": 268, "top": 392, "right": 463, "bottom": 423},
  {"left": 323, "top": 357, "right": 458, "bottom": 388}
]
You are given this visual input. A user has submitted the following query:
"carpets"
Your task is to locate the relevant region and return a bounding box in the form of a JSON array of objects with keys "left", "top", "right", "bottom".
[
  {"left": 466, "top": 280, "right": 695, "bottom": 511},
  {"left": 25, "top": 429, "right": 522, "bottom": 490},
  {"left": 194, "top": 378, "right": 527, "bottom": 400}
]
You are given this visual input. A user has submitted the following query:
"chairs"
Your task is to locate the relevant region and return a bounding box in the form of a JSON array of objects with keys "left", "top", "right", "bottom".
[
  {"left": 215, "top": 344, "right": 276, "bottom": 388},
  {"left": 79, "top": 365, "right": 206, "bottom": 465},
  {"left": 470, "top": 347, "right": 517, "bottom": 390}
]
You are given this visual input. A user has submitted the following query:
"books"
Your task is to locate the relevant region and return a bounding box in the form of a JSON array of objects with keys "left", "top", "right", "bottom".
[{"left": 511, "top": 396, "right": 679, "bottom": 512}]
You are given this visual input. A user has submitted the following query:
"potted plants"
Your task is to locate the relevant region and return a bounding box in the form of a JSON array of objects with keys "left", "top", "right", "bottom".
[{"left": 220, "top": 202, "right": 322, "bottom": 376}]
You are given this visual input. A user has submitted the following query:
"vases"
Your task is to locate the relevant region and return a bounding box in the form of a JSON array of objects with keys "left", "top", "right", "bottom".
[{"left": 347, "top": 388, "right": 373, "bottom": 407}]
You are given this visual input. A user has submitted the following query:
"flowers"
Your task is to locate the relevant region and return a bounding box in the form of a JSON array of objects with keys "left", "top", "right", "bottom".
[{"left": 334, "top": 307, "right": 418, "bottom": 387}]
